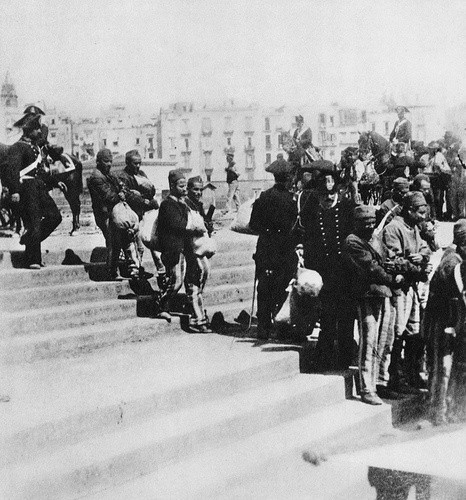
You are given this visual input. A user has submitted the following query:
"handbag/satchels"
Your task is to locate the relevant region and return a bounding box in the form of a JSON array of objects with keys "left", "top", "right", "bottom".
[
  {"left": 133, "top": 174, "right": 156, "bottom": 198},
  {"left": 230, "top": 195, "right": 259, "bottom": 236},
  {"left": 368, "top": 227, "right": 385, "bottom": 258},
  {"left": 184, "top": 210, "right": 207, "bottom": 233},
  {"left": 111, "top": 200, "right": 139, "bottom": 241},
  {"left": 192, "top": 229, "right": 217, "bottom": 255}
]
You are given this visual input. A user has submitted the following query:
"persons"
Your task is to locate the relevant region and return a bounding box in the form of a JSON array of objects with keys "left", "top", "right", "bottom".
[
  {"left": 87, "top": 150, "right": 159, "bottom": 281},
  {"left": 157, "top": 170, "right": 215, "bottom": 332},
  {"left": 7, "top": 106, "right": 68, "bottom": 269},
  {"left": 249, "top": 131, "right": 466, "bottom": 427},
  {"left": 293, "top": 115, "right": 312, "bottom": 167},
  {"left": 388, "top": 106, "right": 412, "bottom": 141},
  {"left": 221, "top": 152, "right": 242, "bottom": 212}
]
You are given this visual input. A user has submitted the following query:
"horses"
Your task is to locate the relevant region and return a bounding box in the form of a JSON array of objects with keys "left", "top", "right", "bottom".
[
  {"left": 279, "top": 130, "right": 322, "bottom": 165},
  {"left": 0, "top": 138, "right": 85, "bottom": 237},
  {"left": 358, "top": 128, "right": 430, "bottom": 181}
]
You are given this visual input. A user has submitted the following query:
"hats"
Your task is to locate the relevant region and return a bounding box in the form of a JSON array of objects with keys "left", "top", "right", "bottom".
[
  {"left": 23, "top": 105, "right": 46, "bottom": 116},
  {"left": 125, "top": 149, "right": 138, "bottom": 158},
  {"left": 168, "top": 169, "right": 186, "bottom": 183},
  {"left": 402, "top": 191, "right": 427, "bottom": 210},
  {"left": 395, "top": 106, "right": 410, "bottom": 112},
  {"left": 12, "top": 112, "right": 41, "bottom": 128},
  {"left": 300, "top": 159, "right": 335, "bottom": 173},
  {"left": 96, "top": 148, "right": 113, "bottom": 161},
  {"left": 353, "top": 205, "right": 377, "bottom": 220},
  {"left": 294, "top": 114, "right": 303, "bottom": 122},
  {"left": 265, "top": 158, "right": 292, "bottom": 173},
  {"left": 453, "top": 218, "right": 466, "bottom": 238},
  {"left": 392, "top": 176, "right": 409, "bottom": 189}
]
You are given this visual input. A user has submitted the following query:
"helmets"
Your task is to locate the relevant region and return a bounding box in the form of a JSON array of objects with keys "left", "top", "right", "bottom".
[{"left": 292, "top": 257, "right": 323, "bottom": 298}]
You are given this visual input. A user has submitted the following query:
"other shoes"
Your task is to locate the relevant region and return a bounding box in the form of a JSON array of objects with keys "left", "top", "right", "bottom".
[
  {"left": 360, "top": 391, "right": 383, "bottom": 406},
  {"left": 157, "top": 311, "right": 172, "bottom": 320},
  {"left": 30, "top": 263, "right": 41, "bottom": 270},
  {"left": 188, "top": 322, "right": 212, "bottom": 333}
]
